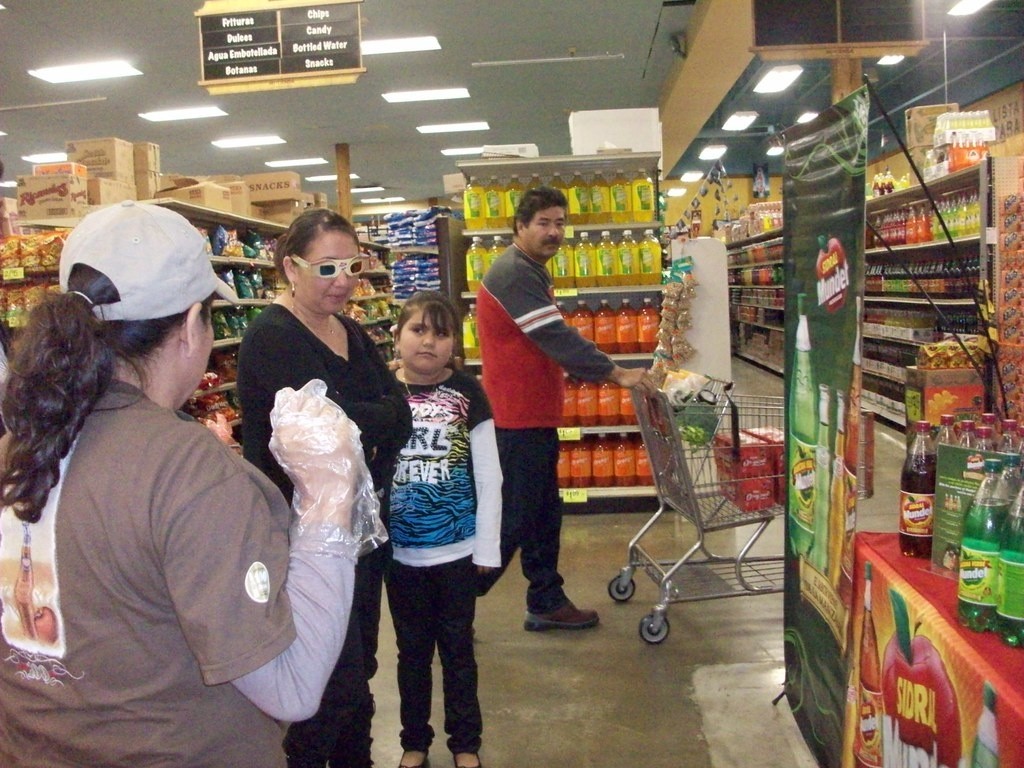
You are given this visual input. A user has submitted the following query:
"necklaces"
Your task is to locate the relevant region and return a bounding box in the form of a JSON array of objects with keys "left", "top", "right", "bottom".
[{"left": 293, "top": 299, "right": 334, "bottom": 335}]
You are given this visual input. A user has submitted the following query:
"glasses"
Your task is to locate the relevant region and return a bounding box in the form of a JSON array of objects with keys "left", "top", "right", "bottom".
[{"left": 291, "top": 254, "right": 363, "bottom": 279}]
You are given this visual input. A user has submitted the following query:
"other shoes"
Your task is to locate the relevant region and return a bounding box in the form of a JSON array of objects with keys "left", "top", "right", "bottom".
[
  {"left": 399, "top": 751, "right": 428, "bottom": 768},
  {"left": 454, "top": 752, "right": 481, "bottom": 768}
]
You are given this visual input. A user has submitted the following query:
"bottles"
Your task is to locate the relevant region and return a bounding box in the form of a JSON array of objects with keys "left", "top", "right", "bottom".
[
  {"left": 899, "top": 413, "right": 1024, "bottom": 647},
  {"left": 463, "top": 167, "right": 661, "bottom": 488},
  {"left": 787, "top": 293, "right": 862, "bottom": 604},
  {"left": 862, "top": 110, "right": 997, "bottom": 403},
  {"left": 852, "top": 561, "right": 884, "bottom": 768}
]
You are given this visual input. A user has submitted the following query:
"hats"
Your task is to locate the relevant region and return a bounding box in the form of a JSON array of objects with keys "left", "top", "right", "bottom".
[{"left": 59, "top": 200, "right": 238, "bottom": 321}]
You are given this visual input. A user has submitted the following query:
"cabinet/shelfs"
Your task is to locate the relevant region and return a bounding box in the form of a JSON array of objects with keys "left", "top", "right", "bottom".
[
  {"left": 719, "top": 160, "right": 989, "bottom": 424},
  {"left": 457, "top": 228, "right": 673, "bottom": 500},
  {"left": 0, "top": 194, "right": 465, "bottom": 436}
]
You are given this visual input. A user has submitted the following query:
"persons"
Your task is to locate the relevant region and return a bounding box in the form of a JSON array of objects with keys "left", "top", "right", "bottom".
[
  {"left": 475, "top": 183, "right": 655, "bottom": 631},
  {"left": 236, "top": 207, "right": 413, "bottom": 768},
  {"left": 385, "top": 290, "right": 504, "bottom": 768},
  {"left": 0, "top": 199, "right": 362, "bottom": 768}
]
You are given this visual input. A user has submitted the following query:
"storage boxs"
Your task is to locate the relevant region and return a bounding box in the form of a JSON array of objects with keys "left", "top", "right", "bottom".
[
  {"left": 16, "top": 137, "right": 329, "bottom": 228},
  {"left": 903, "top": 101, "right": 966, "bottom": 149},
  {"left": 901, "top": 362, "right": 983, "bottom": 436},
  {"left": 906, "top": 145, "right": 934, "bottom": 186}
]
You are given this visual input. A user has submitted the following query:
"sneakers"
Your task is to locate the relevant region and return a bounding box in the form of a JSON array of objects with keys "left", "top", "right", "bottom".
[{"left": 523, "top": 602, "right": 599, "bottom": 631}]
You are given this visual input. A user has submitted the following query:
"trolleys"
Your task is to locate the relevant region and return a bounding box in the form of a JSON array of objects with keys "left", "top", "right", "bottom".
[{"left": 606, "top": 370, "right": 789, "bottom": 641}]
